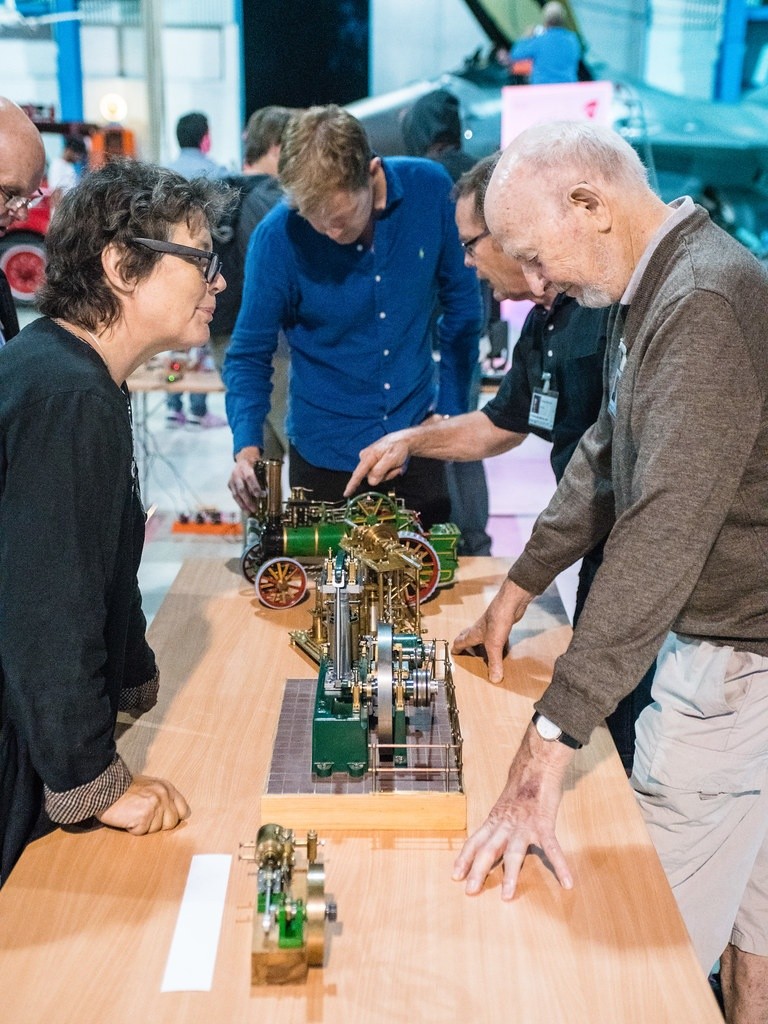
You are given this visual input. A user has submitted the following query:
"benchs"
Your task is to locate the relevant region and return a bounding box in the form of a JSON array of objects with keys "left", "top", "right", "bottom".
[{"left": 120, "top": 371, "right": 229, "bottom": 459}]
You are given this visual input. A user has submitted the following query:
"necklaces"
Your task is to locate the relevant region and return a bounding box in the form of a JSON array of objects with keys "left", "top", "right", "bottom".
[{"left": 84, "top": 328, "right": 136, "bottom": 481}]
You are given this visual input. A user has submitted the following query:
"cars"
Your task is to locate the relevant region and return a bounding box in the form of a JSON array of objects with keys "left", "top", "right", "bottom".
[{"left": 241, "top": 460, "right": 464, "bottom": 612}]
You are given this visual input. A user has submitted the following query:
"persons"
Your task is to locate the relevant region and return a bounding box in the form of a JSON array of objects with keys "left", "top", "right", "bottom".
[
  {"left": 211, "top": 107, "right": 291, "bottom": 519},
  {"left": 220, "top": 105, "right": 483, "bottom": 558},
  {"left": 0, "top": 156, "right": 241, "bottom": 890},
  {"left": 163, "top": 115, "right": 229, "bottom": 431},
  {"left": 401, "top": 91, "right": 491, "bottom": 557},
  {"left": 343, "top": 141, "right": 656, "bottom": 779},
  {"left": 46, "top": 138, "right": 85, "bottom": 229},
  {"left": 508, "top": 2, "right": 588, "bottom": 87},
  {"left": 452, "top": 120, "right": 768, "bottom": 1024},
  {"left": 0, "top": 95, "right": 45, "bottom": 347}
]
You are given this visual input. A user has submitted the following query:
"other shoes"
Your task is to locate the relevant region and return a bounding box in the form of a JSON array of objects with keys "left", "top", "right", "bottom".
[
  {"left": 186, "top": 411, "right": 227, "bottom": 429},
  {"left": 165, "top": 410, "right": 188, "bottom": 425}
]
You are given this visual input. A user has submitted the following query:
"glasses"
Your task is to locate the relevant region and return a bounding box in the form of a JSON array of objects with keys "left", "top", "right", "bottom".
[
  {"left": 460, "top": 230, "right": 491, "bottom": 257},
  {"left": 0, "top": 185, "right": 43, "bottom": 209},
  {"left": 127, "top": 238, "right": 224, "bottom": 284}
]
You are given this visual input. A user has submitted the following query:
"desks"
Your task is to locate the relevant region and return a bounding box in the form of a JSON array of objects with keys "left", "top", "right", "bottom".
[{"left": 0, "top": 556, "right": 727, "bottom": 1023}]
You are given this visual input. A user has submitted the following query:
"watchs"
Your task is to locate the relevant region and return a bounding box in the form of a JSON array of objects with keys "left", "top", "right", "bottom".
[{"left": 532, "top": 712, "right": 584, "bottom": 750}]
